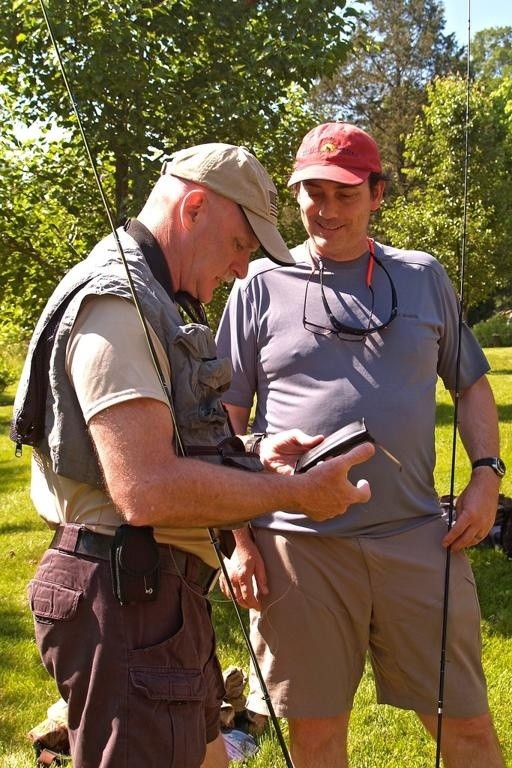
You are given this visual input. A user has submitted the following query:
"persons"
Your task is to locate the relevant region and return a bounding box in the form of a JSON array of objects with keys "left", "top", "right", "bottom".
[
  {"left": 10, "top": 140, "right": 377, "bottom": 766},
  {"left": 212, "top": 121, "right": 507, "bottom": 767}
]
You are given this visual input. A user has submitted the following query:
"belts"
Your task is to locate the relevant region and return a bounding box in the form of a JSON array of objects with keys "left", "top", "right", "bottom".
[{"left": 47, "top": 524, "right": 222, "bottom": 596}]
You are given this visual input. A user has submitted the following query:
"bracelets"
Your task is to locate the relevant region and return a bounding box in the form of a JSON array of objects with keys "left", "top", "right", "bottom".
[{"left": 245, "top": 431, "right": 267, "bottom": 456}]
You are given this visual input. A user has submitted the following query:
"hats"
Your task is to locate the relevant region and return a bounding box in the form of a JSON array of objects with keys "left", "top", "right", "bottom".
[
  {"left": 285, "top": 121, "right": 382, "bottom": 189},
  {"left": 160, "top": 141, "right": 297, "bottom": 269}
]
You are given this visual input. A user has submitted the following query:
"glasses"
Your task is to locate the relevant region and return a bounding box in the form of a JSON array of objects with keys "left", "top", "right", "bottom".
[{"left": 301, "top": 251, "right": 400, "bottom": 344}]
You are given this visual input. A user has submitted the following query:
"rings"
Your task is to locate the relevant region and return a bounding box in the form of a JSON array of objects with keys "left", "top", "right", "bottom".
[{"left": 475, "top": 534, "right": 484, "bottom": 541}]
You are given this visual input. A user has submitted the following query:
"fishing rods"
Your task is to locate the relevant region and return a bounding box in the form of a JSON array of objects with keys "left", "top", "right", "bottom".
[
  {"left": 42, "top": 0, "right": 294, "bottom": 768},
  {"left": 436, "top": 1, "right": 472, "bottom": 768}
]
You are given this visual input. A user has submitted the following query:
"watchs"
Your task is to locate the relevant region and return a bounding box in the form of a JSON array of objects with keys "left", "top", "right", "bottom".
[{"left": 471, "top": 456, "right": 506, "bottom": 480}]
